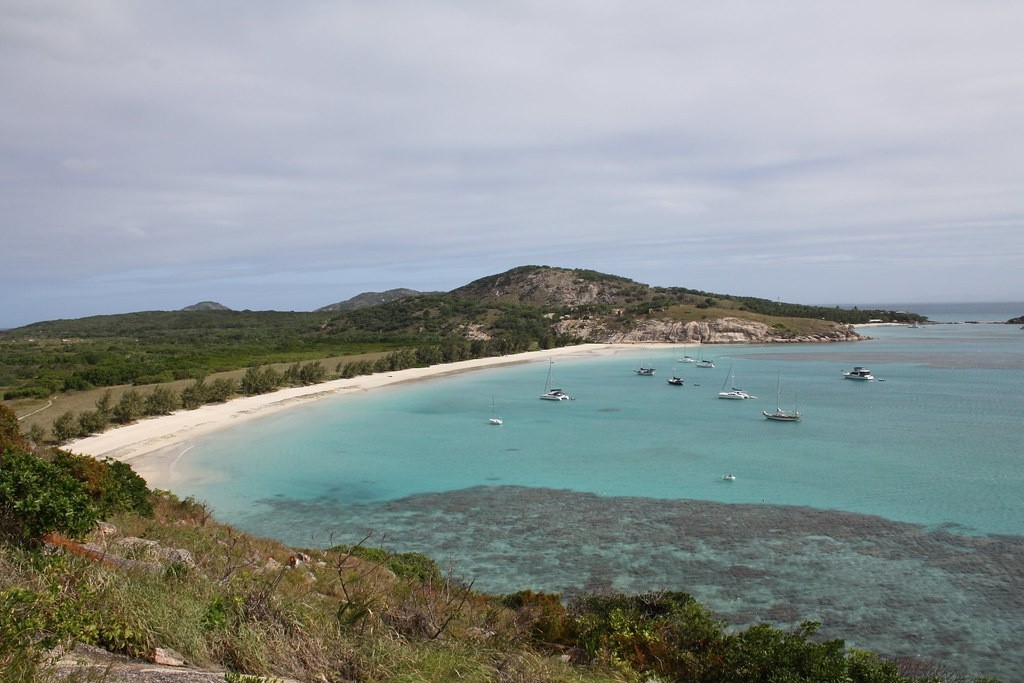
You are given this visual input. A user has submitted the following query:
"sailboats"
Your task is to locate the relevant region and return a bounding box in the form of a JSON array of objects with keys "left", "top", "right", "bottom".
[
  {"left": 697, "top": 339, "right": 716, "bottom": 368},
  {"left": 762, "top": 368, "right": 802, "bottom": 422},
  {"left": 667, "top": 347, "right": 684, "bottom": 385},
  {"left": 717, "top": 357, "right": 752, "bottom": 399},
  {"left": 540, "top": 354, "right": 569, "bottom": 401},
  {"left": 489, "top": 393, "right": 503, "bottom": 425}
]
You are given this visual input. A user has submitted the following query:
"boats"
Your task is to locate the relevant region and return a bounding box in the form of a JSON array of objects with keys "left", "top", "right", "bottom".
[
  {"left": 878, "top": 378, "right": 886, "bottom": 381},
  {"left": 633, "top": 367, "right": 655, "bottom": 376},
  {"left": 677, "top": 356, "right": 695, "bottom": 364},
  {"left": 723, "top": 475, "right": 736, "bottom": 480},
  {"left": 840, "top": 366, "right": 873, "bottom": 380}
]
